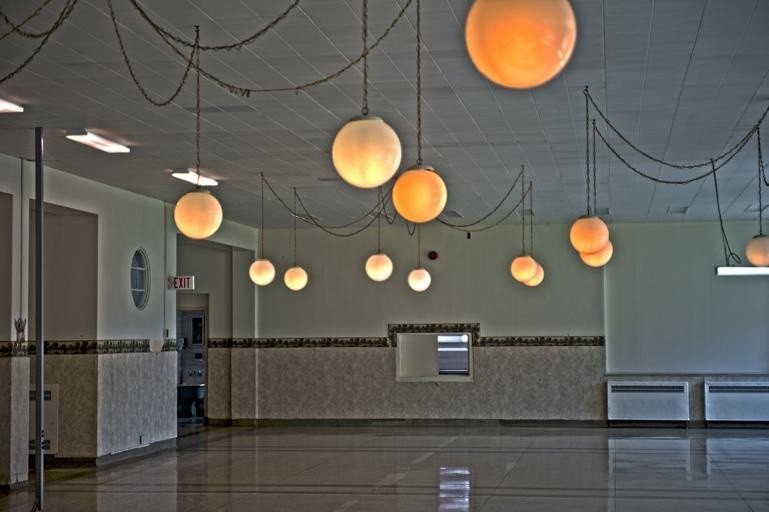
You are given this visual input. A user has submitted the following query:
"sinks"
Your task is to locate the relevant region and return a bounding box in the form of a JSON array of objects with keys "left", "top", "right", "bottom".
[{"left": 180, "top": 383, "right": 205, "bottom": 400}]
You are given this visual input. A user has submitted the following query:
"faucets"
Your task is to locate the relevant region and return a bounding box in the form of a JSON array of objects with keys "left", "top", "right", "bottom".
[{"left": 189, "top": 369, "right": 196, "bottom": 376}]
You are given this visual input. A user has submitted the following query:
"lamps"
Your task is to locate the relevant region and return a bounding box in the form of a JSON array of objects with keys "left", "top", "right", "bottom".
[
  {"left": 173, "top": 22, "right": 311, "bottom": 291},
  {"left": 501, "top": 86, "right": 615, "bottom": 288},
  {"left": 332, "top": 0, "right": 449, "bottom": 292},
  {"left": 463, "top": 0, "right": 579, "bottom": 91},
  {"left": 711, "top": 126, "right": 769, "bottom": 277}
]
